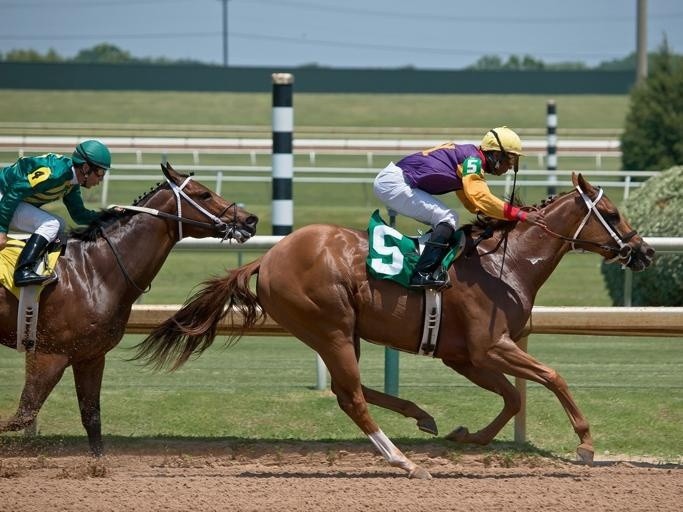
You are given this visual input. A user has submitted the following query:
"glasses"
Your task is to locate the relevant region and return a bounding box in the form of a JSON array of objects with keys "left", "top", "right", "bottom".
[{"left": 93, "top": 167, "right": 105, "bottom": 177}]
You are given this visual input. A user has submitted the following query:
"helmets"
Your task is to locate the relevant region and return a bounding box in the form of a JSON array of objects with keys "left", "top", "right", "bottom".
[
  {"left": 479, "top": 126, "right": 528, "bottom": 160},
  {"left": 69, "top": 140, "right": 112, "bottom": 171}
]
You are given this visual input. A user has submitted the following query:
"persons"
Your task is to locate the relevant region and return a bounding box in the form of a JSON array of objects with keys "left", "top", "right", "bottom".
[
  {"left": 0, "top": 140, "right": 124, "bottom": 286},
  {"left": 373, "top": 125, "right": 546, "bottom": 290}
]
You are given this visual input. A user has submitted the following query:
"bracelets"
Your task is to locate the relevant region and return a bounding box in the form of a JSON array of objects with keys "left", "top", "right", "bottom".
[{"left": 521, "top": 211, "right": 528, "bottom": 221}]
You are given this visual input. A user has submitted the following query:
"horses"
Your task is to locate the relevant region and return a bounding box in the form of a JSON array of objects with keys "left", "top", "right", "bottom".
[
  {"left": 119, "top": 171, "right": 656, "bottom": 479},
  {"left": 0, "top": 162, "right": 259, "bottom": 460}
]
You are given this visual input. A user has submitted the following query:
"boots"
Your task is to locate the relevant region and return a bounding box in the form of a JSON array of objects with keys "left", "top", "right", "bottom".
[
  {"left": 12, "top": 232, "right": 55, "bottom": 288},
  {"left": 408, "top": 221, "right": 457, "bottom": 289}
]
